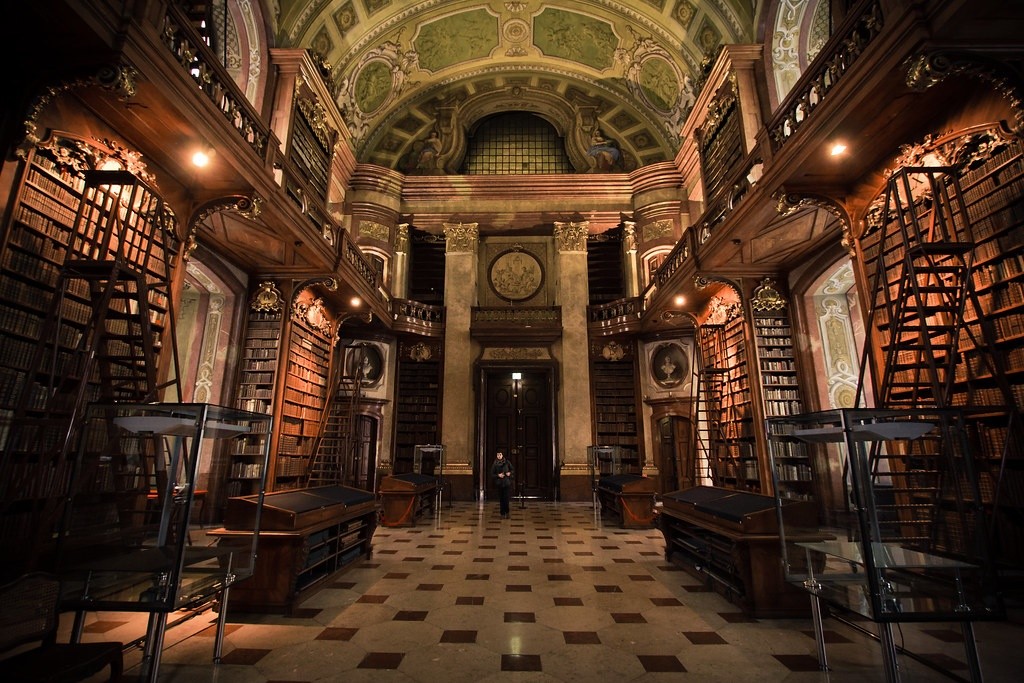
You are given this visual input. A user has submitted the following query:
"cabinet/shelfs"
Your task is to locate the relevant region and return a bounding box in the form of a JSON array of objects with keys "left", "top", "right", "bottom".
[
  {"left": 262, "top": 72, "right": 336, "bottom": 234},
  {"left": 392, "top": 358, "right": 441, "bottom": 474},
  {"left": 223, "top": 287, "right": 338, "bottom": 523},
  {"left": 700, "top": 311, "right": 820, "bottom": 528},
  {"left": 592, "top": 361, "right": 640, "bottom": 460},
  {"left": 696, "top": 70, "right": 763, "bottom": 228}
]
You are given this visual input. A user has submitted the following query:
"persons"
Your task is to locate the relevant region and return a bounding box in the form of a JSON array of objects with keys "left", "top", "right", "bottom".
[{"left": 491, "top": 447, "right": 516, "bottom": 519}]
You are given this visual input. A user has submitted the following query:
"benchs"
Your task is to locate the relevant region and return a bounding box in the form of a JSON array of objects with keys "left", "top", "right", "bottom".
[{"left": 0, "top": 570, "right": 125, "bottom": 683}]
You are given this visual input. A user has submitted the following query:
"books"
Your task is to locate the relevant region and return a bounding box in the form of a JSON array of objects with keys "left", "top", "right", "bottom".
[
  {"left": 702, "top": 315, "right": 816, "bottom": 504},
  {"left": 0, "top": 153, "right": 180, "bottom": 594},
  {"left": 701, "top": 106, "right": 752, "bottom": 211},
  {"left": 592, "top": 369, "right": 641, "bottom": 477},
  {"left": 291, "top": 104, "right": 331, "bottom": 205},
  {"left": 217, "top": 311, "right": 333, "bottom": 516},
  {"left": 861, "top": 137, "right": 1024, "bottom": 606},
  {"left": 395, "top": 361, "right": 443, "bottom": 474}
]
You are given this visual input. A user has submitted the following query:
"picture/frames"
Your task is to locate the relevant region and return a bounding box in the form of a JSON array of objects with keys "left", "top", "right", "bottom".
[{"left": 486, "top": 249, "right": 546, "bottom": 302}]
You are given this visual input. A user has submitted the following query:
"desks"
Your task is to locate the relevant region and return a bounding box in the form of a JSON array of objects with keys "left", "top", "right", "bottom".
[
  {"left": 62, "top": 401, "right": 276, "bottom": 683},
  {"left": 771, "top": 404, "right": 1017, "bottom": 683}
]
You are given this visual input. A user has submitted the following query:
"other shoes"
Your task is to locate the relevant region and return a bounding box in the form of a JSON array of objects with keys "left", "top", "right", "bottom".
[
  {"left": 506, "top": 514, "right": 510, "bottom": 518},
  {"left": 500, "top": 516, "right": 505, "bottom": 519}
]
